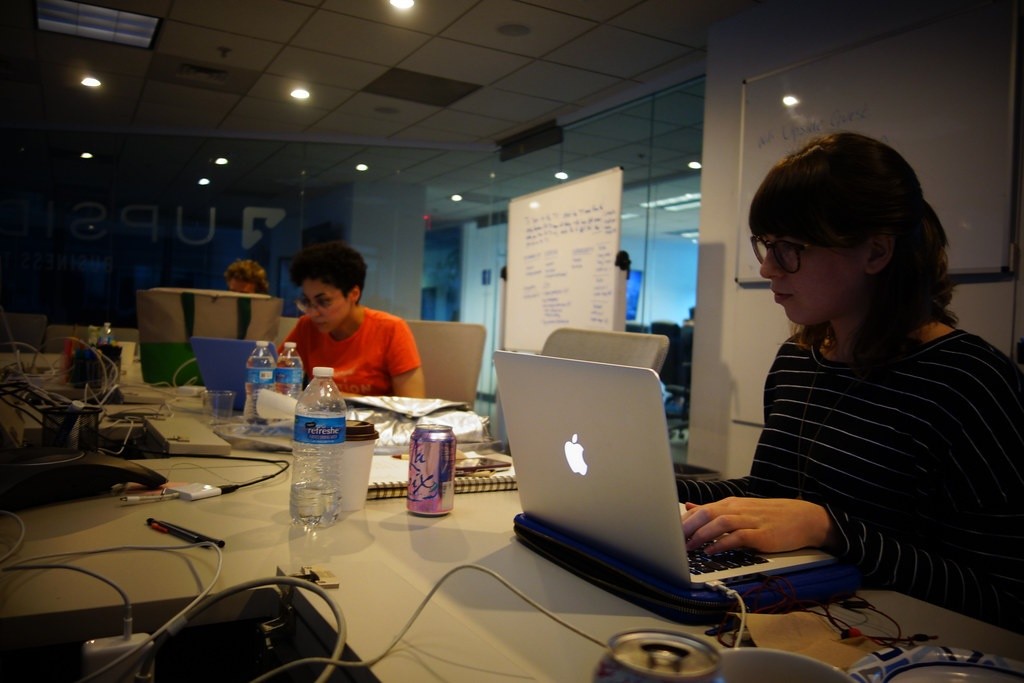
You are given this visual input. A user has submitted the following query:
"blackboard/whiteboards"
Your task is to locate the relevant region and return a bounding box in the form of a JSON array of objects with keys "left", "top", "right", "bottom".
[
  {"left": 735, "top": 0, "right": 1021, "bottom": 285},
  {"left": 503, "top": 165, "right": 623, "bottom": 353}
]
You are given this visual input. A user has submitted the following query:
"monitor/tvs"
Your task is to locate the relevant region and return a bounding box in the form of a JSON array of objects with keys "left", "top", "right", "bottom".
[{"left": 302, "top": 223, "right": 332, "bottom": 249}]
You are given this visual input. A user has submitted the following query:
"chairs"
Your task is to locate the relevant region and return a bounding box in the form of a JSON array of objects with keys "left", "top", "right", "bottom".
[
  {"left": 540, "top": 324, "right": 669, "bottom": 374},
  {"left": 404, "top": 318, "right": 487, "bottom": 408}
]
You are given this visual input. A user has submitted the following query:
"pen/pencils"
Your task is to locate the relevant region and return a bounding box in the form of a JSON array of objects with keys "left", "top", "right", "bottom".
[
  {"left": 159, "top": 521, "right": 225, "bottom": 548},
  {"left": 146, "top": 517, "right": 210, "bottom": 548},
  {"left": 392, "top": 454, "right": 409, "bottom": 459}
]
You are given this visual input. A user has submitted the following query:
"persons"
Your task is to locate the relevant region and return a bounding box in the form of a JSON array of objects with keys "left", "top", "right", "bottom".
[
  {"left": 672, "top": 132, "right": 1023, "bottom": 633},
  {"left": 226, "top": 260, "right": 268, "bottom": 296},
  {"left": 274, "top": 240, "right": 425, "bottom": 407}
]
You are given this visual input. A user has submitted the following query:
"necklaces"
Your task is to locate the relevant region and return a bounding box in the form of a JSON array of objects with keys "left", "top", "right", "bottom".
[{"left": 791, "top": 327, "right": 889, "bottom": 502}]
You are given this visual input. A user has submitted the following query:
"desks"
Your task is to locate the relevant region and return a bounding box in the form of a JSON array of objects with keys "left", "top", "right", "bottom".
[{"left": 1, "top": 349, "right": 1024, "bottom": 683}]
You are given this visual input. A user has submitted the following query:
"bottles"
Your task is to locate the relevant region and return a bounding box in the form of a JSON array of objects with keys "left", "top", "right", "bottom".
[
  {"left": 276, "top": 341, "right": 303, "bottom": 400},
  {"left": 98, "top": 322, "right": 115, "bottom": 345},
  {"left": 288, "top": 366, "right": 347, "bottom": 529},
  {"left": 242, "top": 340, "right": 278, "bottom": 425}
]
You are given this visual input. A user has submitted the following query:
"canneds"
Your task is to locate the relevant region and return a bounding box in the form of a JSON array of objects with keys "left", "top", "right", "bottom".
[
  {"left": 590, "top": 627, "right": 725, "bottom": 683},
  {"left": 406, "top": 424, "right": 457, "bottom": 516}
]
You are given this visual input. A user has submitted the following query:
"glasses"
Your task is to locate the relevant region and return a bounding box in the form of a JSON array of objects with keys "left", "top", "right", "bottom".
[
  {"left": 295, "top": 296, "right": 347, "bottom": 312},
  {"left": 751, "top": 235, "right": 859, "bottom": 273}
]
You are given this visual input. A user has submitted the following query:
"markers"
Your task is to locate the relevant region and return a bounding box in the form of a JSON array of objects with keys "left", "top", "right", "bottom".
[{"left": 52, "top": 400, "right": 85, "bottom": 448}]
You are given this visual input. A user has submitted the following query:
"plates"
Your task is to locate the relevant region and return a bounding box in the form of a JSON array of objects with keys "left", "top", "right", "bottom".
[{"left": 847, "top": 644, "right": 1024, "bottom": 683}]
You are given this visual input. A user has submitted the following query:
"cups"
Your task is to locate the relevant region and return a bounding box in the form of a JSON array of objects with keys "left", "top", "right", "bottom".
[
  {"left": 201, "top": 390, "right": 234, "bottom": 428},
  {"left": 341, "top": 419, "right": 380, "bottom": 513}
]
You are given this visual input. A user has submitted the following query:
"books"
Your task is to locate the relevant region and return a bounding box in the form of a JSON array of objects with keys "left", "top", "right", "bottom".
[{"left": 367, "top": 444, "right": 519, "bottom": 499}]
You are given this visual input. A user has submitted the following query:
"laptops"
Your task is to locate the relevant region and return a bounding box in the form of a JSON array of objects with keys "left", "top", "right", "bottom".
[{"left": 492, "top": 350, "right": 837, "bottom": 587}]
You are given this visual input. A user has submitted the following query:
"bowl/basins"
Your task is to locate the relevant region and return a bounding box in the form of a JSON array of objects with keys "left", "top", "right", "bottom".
[{"left": 712, "top": 647, "right": 861, "bottom": 683}]
